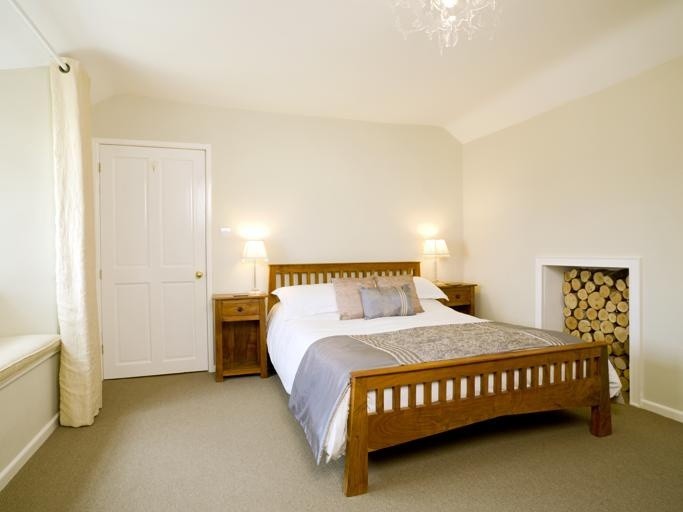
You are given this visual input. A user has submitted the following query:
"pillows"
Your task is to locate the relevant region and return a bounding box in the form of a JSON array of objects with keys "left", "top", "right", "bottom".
[{"left": 271, "top": 274, "right": 448, "bottom": 320}]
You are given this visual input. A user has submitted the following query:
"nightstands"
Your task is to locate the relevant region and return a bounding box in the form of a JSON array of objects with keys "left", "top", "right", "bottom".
[
  {"left": 434, "top": 281, "right": 477, "bottom": 316},
  {"left": 213, "top": 292, "right": 268, "bottom": 382}
]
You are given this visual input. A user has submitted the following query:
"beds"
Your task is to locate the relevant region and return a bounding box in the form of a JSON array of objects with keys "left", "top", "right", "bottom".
[{"left": 267, "top": 262, "right": 623, "bottom": 496}]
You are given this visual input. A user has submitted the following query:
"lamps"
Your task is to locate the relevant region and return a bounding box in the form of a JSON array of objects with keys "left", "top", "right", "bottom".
[
  {"left": 392, "top": 0, "right": 497, "bottom": 54},
  {"left": 423, "top": 239, "right": 451, "bottom": 280},
  {"left": 241, "top": 240, "right": 269, "bottom": 290}
]
